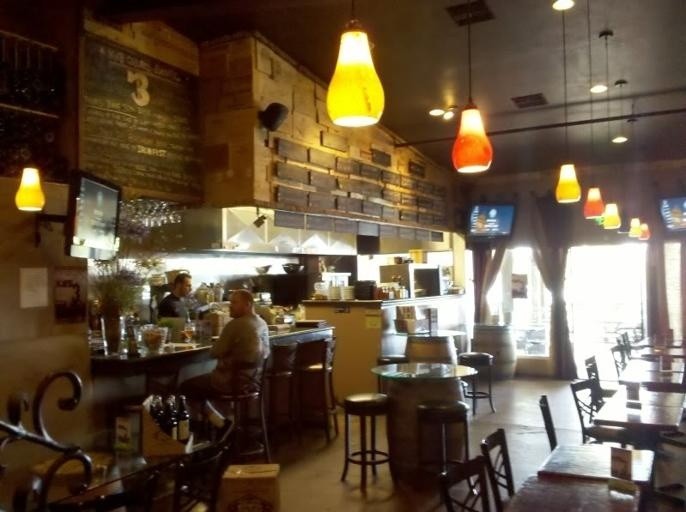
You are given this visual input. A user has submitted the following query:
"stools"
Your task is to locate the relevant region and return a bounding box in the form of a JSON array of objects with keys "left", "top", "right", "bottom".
[
  {"left": 458, "top": 351, "right": 496, "bottom": 415},
  {"left": 376, "top": 354, "right": 409, "bottom": 393},
  {"left": 339, "top": 391, "right": 392, "bottom": 495},
  {"left": 413, "top": 397, "right": 478, "bottom": 511}
]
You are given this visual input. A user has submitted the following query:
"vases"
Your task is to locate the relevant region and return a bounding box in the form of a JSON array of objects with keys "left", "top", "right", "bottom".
[{"left": 99, "top": 312, "right": 124, "bottom": 355}]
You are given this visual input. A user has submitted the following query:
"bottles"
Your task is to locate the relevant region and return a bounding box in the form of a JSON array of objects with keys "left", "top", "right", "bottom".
[
  {"left": 87, "top": 298, "right": 107, "bottom": 354},
  {"left": 150, "top": 395, "right": 189, "bottom": 444}
]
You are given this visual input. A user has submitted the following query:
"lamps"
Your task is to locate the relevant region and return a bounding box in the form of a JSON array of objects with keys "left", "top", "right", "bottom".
[
  {"left": 597, "top": 28, "right": 652, "bottom": 243},
  {"left": 550, "top": 0, "right": 581, "bottom": 204},
  {"left": 451, "top": 1, "right": 495, "bottom": 173},
  {"left": 326, "top": 0, "right": 385, "bottom": 129},
  {"left": 578, "top": 1, "right": 605, "bottom": 221},
  {"left": 14, "top": 167, "right": 44, "bottom": 215}
]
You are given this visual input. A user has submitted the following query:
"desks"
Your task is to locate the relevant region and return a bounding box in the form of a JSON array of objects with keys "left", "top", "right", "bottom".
[
  {"left": 535, "top": 442, "right": 655, "bottom": 483},
  {"left": 501, "top": 475, "right": 642, "bottom": 512},
  {"left": 590, "top": 335, "right": 685, "bottom": 432},
  {"left": 369, "top": 361, "right": 478, "bottom": 481}
]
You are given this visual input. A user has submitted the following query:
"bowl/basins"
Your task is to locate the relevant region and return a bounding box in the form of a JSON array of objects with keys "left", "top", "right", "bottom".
[
  {"left": 394, "top": 256, "right": 405, "bottom": 264},
  {"left": 284, "top": 264, "right": 304, "bottom": 274}
]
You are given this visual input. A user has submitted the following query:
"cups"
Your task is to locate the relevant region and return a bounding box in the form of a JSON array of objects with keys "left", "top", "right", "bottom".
[
  {"left": 143, "top": 326, "right": 169, "bottom": 354},
  {"left": 184, "top": 323, "right": 196, "bottom": 339}
]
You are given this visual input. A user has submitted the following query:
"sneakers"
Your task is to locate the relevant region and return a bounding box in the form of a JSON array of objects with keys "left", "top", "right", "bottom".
[{"left": 218, "top": 418, "right": 235, "bottom": 444}]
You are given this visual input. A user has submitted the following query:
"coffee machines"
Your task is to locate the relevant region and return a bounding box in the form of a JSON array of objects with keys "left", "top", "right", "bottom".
[{"left": 149, "top": 286, "right": 173, "bottom": 319}]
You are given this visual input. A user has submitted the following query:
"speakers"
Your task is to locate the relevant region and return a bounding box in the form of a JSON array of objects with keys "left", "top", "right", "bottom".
[{"left": 261, "top": 103, "right": 289, "bottom": 131}]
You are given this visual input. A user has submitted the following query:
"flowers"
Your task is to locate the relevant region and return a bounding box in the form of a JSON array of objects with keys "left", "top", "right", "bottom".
[{"left": 88, "top": 219, "right": 166, "bottom": 344}]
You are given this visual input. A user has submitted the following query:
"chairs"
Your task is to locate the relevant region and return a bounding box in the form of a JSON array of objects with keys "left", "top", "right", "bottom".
[
  {"left": 583, "top": 334, "right": 632, "bottom": 404},
  {"left": 538, "top": 394, "right": 557, "bottom": 449},
  {"left": 569, "top": 377, "right": 601, "bottom": 444},
  {"left": 434, "top": 454, "right": 492, "bottom": 512},
  {"left": 209, "top": 350, "right": 272, "bottom": 467},
  {"left": 274, "top": 337, "right": 340, "bottom": 443},
  {"left": 480, "top": 428, "right": 516, "bottom": 506}
]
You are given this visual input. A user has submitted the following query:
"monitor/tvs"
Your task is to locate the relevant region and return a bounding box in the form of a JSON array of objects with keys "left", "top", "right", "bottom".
[
  {"left": 657, "top": 194, "right": 686, "bottom": 234},
  {"left": 465, "top": 201, "right": 517, "bottom": 243},
  {"left": 65, "top": 169, "right": 122, "bottom": 262}
]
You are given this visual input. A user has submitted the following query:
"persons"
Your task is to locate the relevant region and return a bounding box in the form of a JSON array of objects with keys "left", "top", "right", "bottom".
[
  {"left": 158, "top": 274, "right": 192, "bottom": 324},
  {"left": 179, "top": 289, "right": 271, "bottom": 438}
]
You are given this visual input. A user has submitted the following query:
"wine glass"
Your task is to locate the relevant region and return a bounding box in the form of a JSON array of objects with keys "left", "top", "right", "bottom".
[{"left": 121, "top": 195, "right": 183, "bottom": 229}]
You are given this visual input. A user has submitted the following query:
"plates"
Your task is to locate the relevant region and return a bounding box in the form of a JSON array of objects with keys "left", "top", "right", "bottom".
[
  {"left": 329, "top": 287, "right": 354, "bottom": 299},
  {"left": 355, "top": 280, "right": 377, "bottom": 300}
]
